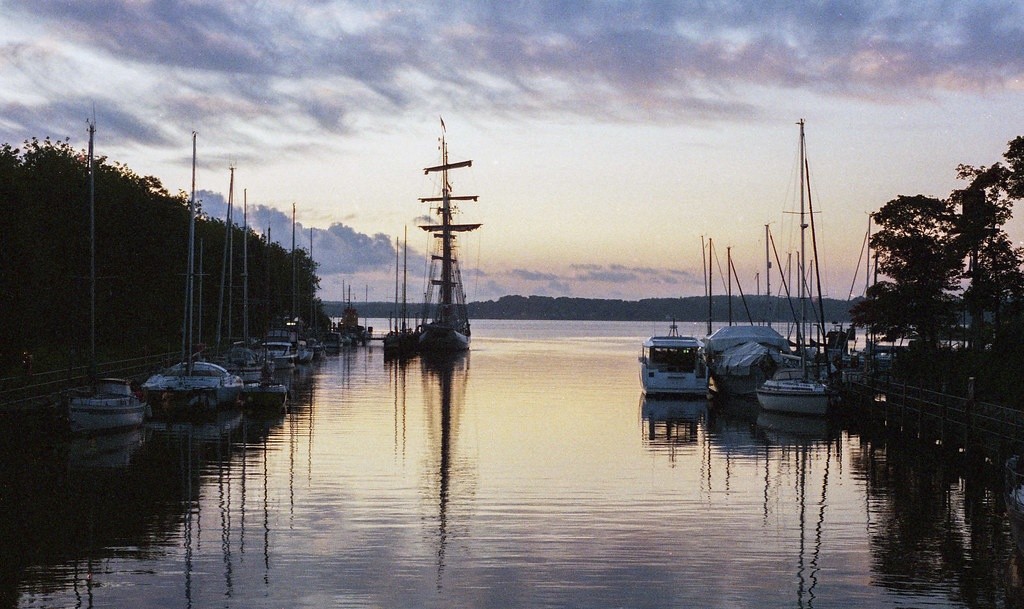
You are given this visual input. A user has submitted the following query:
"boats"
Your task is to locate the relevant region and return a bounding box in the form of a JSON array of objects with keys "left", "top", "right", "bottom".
[
  {"left": 639, "top": 391, "right": 709, "bottom": 463},
  {"left": 637, "top": 333, "right": 709, "bottom": 399}
]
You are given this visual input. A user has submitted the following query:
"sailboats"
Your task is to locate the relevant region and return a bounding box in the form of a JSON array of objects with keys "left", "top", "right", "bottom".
[
  {"left": 65, "top": 120, "right": 484, "bottom": 436},
  {"left": 701, "top": 117, "right": 907, "bottom": 415},
  {"left": 757, "top": 412, "right": 836, "bottom": 609}
]
